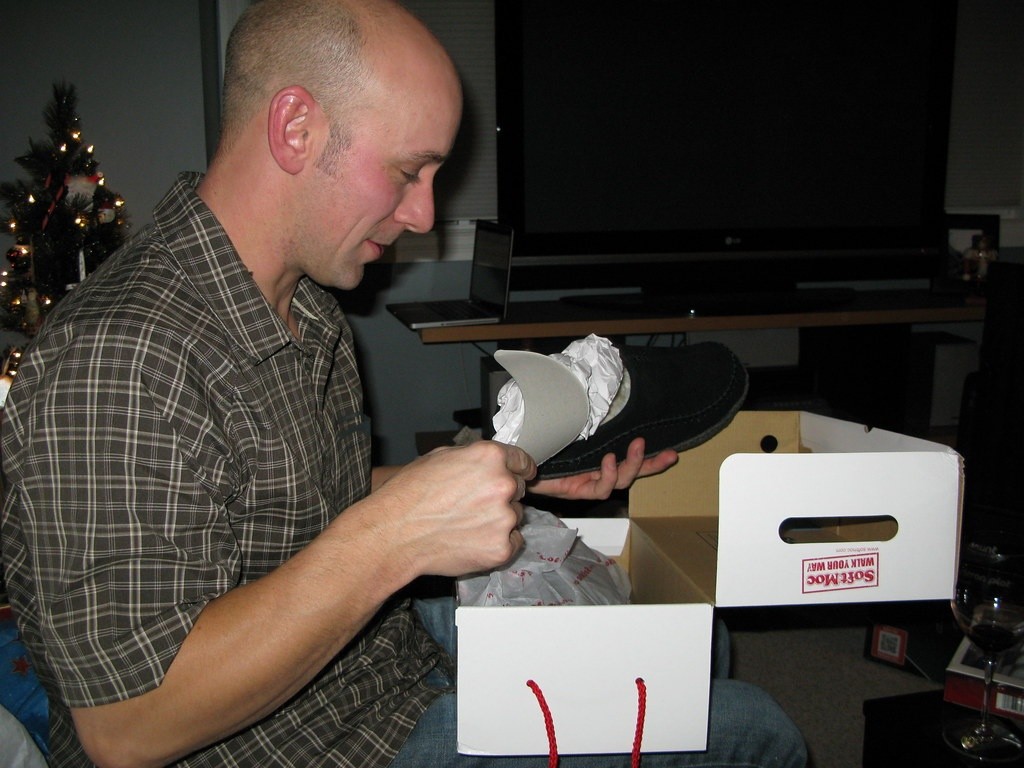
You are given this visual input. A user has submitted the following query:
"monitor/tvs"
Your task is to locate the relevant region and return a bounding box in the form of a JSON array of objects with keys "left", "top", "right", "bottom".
[{"left": 493, "top": 0, "right": 960, "bottom": 305}]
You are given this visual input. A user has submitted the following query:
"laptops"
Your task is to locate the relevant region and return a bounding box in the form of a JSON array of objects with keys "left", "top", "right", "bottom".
[{"left": 385, "top": 219, "right": 513, "bottom": 329}]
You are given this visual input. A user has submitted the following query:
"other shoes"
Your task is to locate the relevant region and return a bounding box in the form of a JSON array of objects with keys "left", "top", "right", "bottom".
[{"left": 489, "top": 341, "right": 748, "bottom": 481}]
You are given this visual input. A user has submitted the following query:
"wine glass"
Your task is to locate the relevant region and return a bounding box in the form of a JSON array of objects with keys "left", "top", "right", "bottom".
[{"left": 942, "top": 529, "right": 1024, "bottom": 762}]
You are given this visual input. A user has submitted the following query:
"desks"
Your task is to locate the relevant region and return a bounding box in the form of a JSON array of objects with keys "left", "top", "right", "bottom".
[{"left": 387, "top": 290, "right": 987, "bottom": 459}]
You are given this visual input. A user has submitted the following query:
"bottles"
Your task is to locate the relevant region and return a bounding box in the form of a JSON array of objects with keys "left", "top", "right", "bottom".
[{"left": 962, "top": 235, "right": 988, "bottom": 281}]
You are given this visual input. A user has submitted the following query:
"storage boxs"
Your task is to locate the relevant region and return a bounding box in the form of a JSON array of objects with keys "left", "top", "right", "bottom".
[{"left": 454, "top": 409, "right": 964, "bottom": 768}]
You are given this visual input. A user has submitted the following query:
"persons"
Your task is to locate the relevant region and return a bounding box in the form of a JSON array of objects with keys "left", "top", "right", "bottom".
[{"left": 2, "top": 3, "right": 811, "bottom": 766}]
